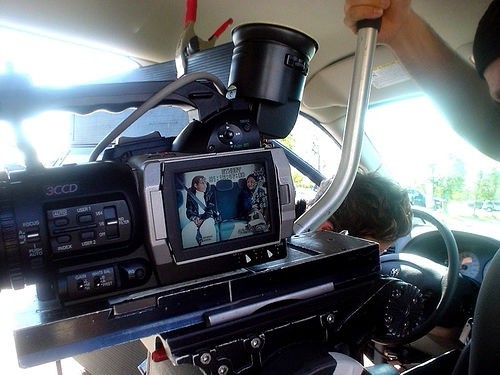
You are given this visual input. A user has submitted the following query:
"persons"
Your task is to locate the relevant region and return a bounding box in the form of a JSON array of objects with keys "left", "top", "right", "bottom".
[
  {"left": 180, "top": 174, "right": 268, "bottom": 250},
  {"left": 342, "top": 1, "right": 500, "bottom": 375},
  {"left": 295, "top": 170, "right": 412, "bottom": 256}
]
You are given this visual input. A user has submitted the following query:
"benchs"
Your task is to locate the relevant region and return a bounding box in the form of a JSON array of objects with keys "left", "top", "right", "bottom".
[{"left": 176, "top": 174, "right": 268, "bottom": 243}]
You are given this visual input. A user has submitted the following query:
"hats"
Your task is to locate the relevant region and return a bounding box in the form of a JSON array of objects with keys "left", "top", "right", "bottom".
[{"left": 472, "top": 0, "right": 500, "bottom": 79}]
